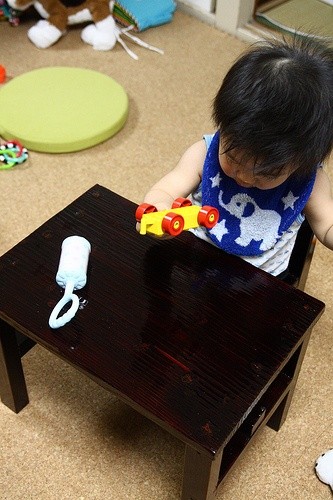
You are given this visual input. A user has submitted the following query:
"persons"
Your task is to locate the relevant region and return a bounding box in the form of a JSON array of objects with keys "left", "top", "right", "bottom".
[{"left": 136, "top": 27, "right": 333, "bottom": 277}]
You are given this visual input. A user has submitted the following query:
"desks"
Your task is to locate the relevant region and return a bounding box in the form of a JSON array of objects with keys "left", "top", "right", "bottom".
[{"left": 0, "top": 184, "right": 326, "bottom": 500}]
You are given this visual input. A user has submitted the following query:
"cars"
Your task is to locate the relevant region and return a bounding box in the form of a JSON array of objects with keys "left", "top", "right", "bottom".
[{"left": 136, "top": 199, "right": 220, "bottom": 236}]
